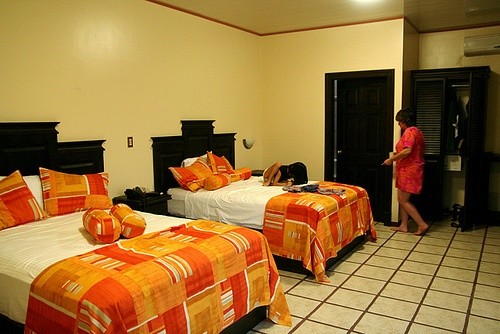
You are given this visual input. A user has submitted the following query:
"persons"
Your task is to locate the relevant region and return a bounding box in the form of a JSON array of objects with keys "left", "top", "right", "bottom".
[
  {"left": 263, "top": 160, "right": 307, "bottom": 187},
  {"left": 383, "top": 106, "right": 430, "bottom": 236}
]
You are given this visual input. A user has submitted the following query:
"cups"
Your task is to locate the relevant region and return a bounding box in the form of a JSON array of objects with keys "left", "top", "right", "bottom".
[{"left": 390, "top": 152, "right": 395, "bottom": 158}]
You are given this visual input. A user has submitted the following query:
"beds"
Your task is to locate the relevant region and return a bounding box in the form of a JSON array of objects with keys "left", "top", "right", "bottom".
[
  {"left": 0, "top": 122, "right": 267, "bottom": 331},
  {"left": 151, "top": 118, "right": 336, "bottom": 275}
]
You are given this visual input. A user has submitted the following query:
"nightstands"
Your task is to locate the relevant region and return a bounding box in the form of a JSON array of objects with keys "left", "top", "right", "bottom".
[
  {"left": 248, "top": 167, "right": 266, "bottom": 178},
  {"left": 112, "top": 190, "right": 173, "bottom": 218}
]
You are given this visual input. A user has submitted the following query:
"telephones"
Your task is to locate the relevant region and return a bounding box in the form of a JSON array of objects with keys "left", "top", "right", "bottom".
[{"left": 124, "top": 189, "right": 146, "bottom": 201}]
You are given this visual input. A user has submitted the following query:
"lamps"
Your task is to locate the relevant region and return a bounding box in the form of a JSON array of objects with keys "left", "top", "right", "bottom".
[{"left": 242, "top": 138, "right": 260, "bottom": 150}]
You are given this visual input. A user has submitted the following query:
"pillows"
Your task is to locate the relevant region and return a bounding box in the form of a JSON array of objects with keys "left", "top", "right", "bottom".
[
  {"left": 17, "top": 165, "right": 46, "bottom": 220},
  {"left": 182, "top": 149, "right": 213, "bottom": 168},
  {"left": 0, "top": 166, "right": 51, "bottom": 234},
  {"left": 168, "top": 157, "right": 211, "bottom": 191},
  {"left": 204, "top": 151, "right": 233, "bottom": 174},
  {"left": 109, "top": 200, "right": 147, "bottom": 239},
  {"left": 39, "top": 164, "right": 112, "bottom": 216},
  {"left": 82, "top": 203, "right": 120, "bottom": 243},
  {"left": 209, "top": 165, "right": 252, "bottom": 191}
]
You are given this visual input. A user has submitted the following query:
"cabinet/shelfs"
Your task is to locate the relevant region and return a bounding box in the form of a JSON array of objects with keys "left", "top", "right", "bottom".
[{"left": 409, "top": 65, "right": 488, "bottom": 233}]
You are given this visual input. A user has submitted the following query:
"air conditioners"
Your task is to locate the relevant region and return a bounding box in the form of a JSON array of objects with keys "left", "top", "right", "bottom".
[{"left": 463, "top": 35, "right": 500, "bottom": 57}]
